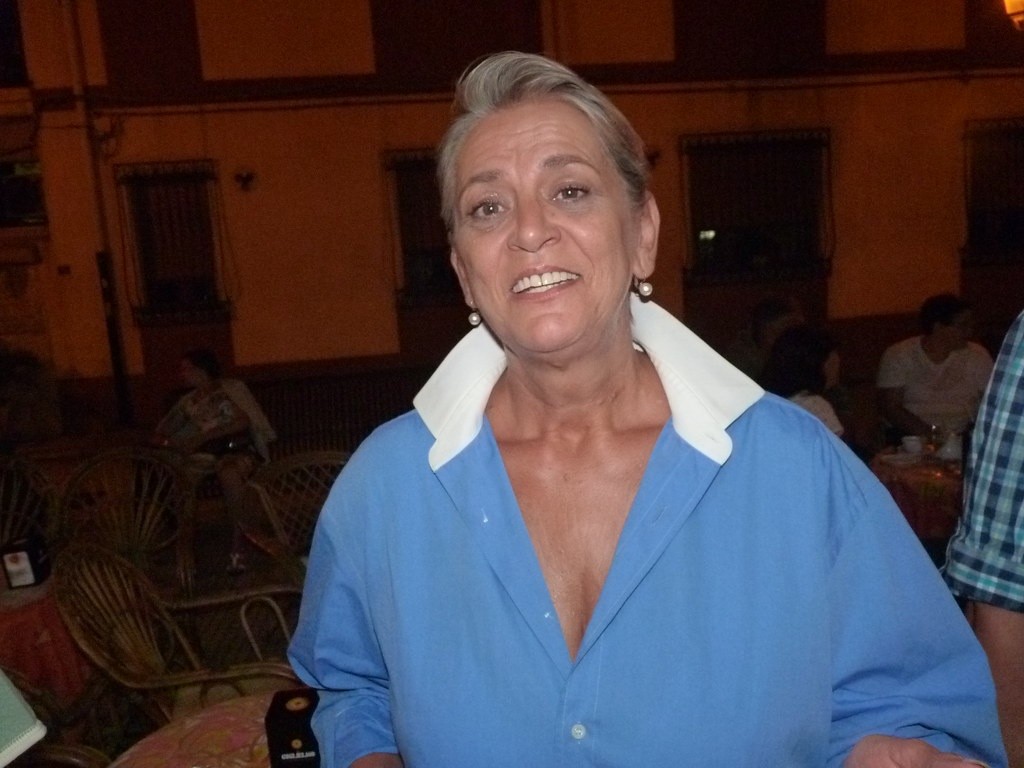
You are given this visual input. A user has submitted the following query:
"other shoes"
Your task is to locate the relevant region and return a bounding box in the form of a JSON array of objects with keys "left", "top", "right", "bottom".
[
  {"left": 169, "top": 567, "right": 194, "bottom": 593},
  {"left": 227, "top": 547, "right": 249, "bottom": 575}
]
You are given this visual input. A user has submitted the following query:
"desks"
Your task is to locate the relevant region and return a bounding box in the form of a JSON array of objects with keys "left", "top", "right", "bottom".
[
  {"left": 0, "top": 562, "right": 107, "bottom": 727},
  {"left": 868, "top": 444, "right": 963, "bottom": 540},
  {"left": 103, "top": 691, "right": 277, "bottom": 768}
]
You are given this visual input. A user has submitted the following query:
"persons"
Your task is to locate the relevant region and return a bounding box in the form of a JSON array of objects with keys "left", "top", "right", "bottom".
[
  {"left": 753, "top": 291, "right": 1024, "bottom": 768},
  {"left": 155, "top": 349, "right": 276, "bottom": 576},
  {"left": 287, "top": 51, "right": 1008, "bottom": 768}
]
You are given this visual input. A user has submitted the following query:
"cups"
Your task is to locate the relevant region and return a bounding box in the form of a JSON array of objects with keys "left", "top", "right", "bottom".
[{"left": 918, "top": 447, "right": 936, "bottom": 476}]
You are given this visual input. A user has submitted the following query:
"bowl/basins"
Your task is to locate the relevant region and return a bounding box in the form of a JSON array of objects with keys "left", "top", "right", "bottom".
[{"left": 902, "top": 436, "right": 921, "bottom": 453}]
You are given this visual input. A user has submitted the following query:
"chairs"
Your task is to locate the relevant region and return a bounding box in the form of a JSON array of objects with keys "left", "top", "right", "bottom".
[{"left": 0, "top": 447, "right": 349, "bottom": 768}]
